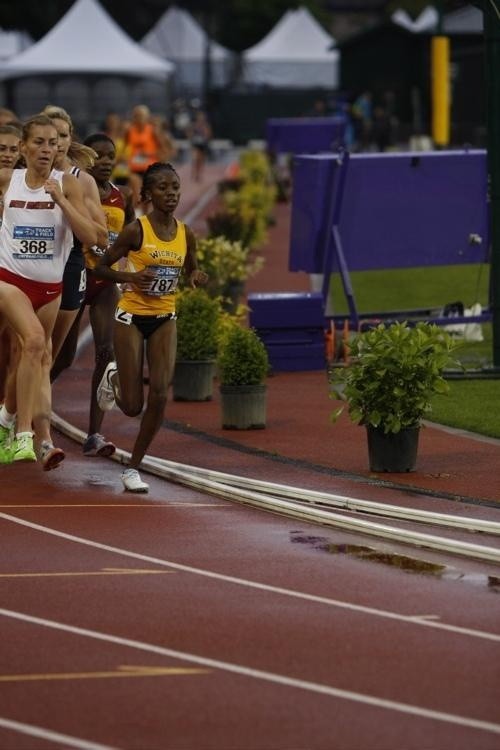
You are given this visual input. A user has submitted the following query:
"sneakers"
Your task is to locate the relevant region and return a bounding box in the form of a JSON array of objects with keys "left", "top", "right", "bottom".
[
  {"left": 0, "top": 406, "right": 65, "bottom": 472},
  {"left": 84, "top": 433, "right": 115, "bottom": 456},
  {"left": 120, "top": 468, "right": 149, "bottom": 492},
  {"left": 97, "top": 363, "right": 118, "bottom": 411}
]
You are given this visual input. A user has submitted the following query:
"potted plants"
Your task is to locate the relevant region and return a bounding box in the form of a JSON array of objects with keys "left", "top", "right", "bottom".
[
  {"left": 216, "top": 321, "right": 270, "bottom": 432},
  {"left": 172, "top": 289, "right": 253, "bottom": 403},
  {"left": 328, "top": 321, "right": 462, "bottom": 473},
  {"left": 196, "top": 152, "right": 280, "bottom": 315}
]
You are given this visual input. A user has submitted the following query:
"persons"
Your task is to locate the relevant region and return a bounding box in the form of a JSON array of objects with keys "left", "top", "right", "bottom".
[
  {"left": 307, "top": 89, "right": 397, "bottom": 147},
  {"left": 94, "top": 163, "right": 206, "bottom": 491},
  {"left": 102, "top": 103, "right": 207, "bottom": 214},
  {"left": 0, "top": 105, "right": 137, "bottom": 470}
]
